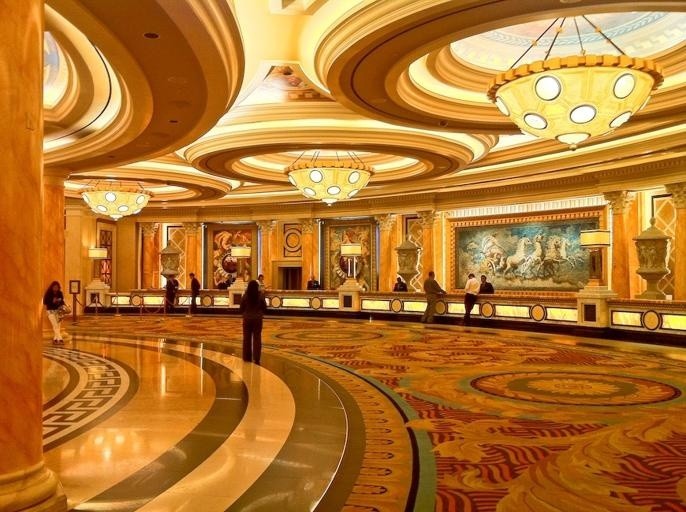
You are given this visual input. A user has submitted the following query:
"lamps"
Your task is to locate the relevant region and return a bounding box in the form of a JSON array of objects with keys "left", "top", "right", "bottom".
[
  {"left": 284, "top": 150, "right": 376, "bottom": 208},
  {"left": 230, "top": 246, "right": 251, "bottom": 276},
  {"left": 486, "top": 15, "right": 666, "bottom": 152},
  {"left": 87, "top": 247, "right": 108, "bottom": 280},
  {"left": 77, "top": 179, "right": 155, "bottom": 222},
  {"left": 580, "top": 229, "right": 611, "bottom": 278},
  {"left": 339, "top": 243, "right": 362, "bottom": 280}
]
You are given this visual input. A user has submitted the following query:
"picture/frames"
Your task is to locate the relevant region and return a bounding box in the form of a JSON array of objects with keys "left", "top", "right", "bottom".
[
  {"left": 207, "top": 223, "right": 258, "bottom": 288},
  {"left": 447, "top": 211, "right": 609, "bottom": 301},
  {"left": 325, "top": 219, "right": 378, "bottom": 291}
]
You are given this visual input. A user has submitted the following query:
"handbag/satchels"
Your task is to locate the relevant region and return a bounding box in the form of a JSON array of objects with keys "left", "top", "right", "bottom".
[{"left": 57, "top": 303, "right": 72, "bottom": 315}]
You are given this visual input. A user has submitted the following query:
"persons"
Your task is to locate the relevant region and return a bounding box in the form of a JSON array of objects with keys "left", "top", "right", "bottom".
[
  {"left": 479, "top": 275, "right": 494, "bottom": 293},
  {"left": 256, "top": 274, "right": 265, "bottom": 289},
  {"left": 307, "top": 275, "right": 320, "bottom": 290},
  {"left": 421, "top": 271, "right": 445, "bottom": 323},
  {"left": 190, "top": 272, "right": 199, "bottom": 313},
  {"left": 44, "top": 281, "right": 64, "bottom": 345},
  {"left": 395, "top": 277, "right": 411, "bottom": 292},
  {"left": 240, "top": 280, "right": 266, "bottom": 364},
  {"left": 461, "top": 273, "right": 480, "bottom": 324},
  {"left": 168, "top": 275, "right": 179, "bottom": 313}
]
